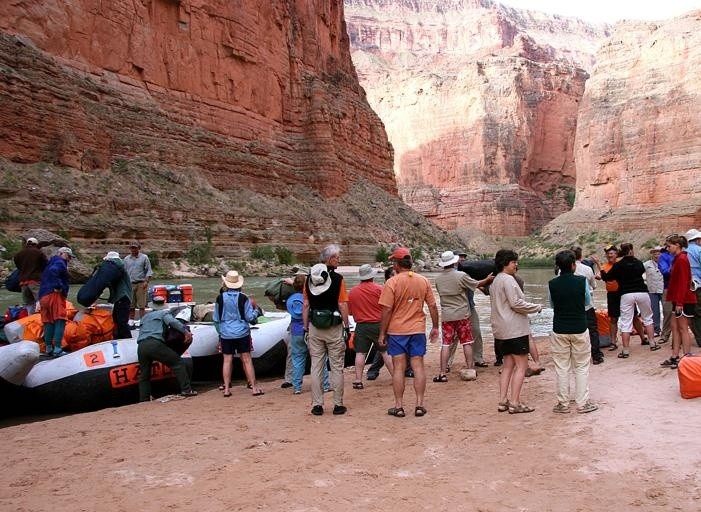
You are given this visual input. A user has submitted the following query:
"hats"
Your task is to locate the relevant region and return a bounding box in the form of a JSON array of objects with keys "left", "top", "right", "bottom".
[
  {"left": 604, "top": 245, "right": 616, "bottom": 252},
  {"left": 148, "top": 297, "right": 169, "bottom": 310},
  {"left": 683, "top": 229, "right": 701, "bottom": 241},
  {"left": 650, "top": 246, "right": 665, "bottom": 253},
  {"left": 129, "top": 240, "right": 141, "bottom": 249},
  {"left": 103, "top": 251, "right": 122, "bottom": 261},
  {"left": 221, "top": 247, "right": 467, "bottom": 295},
  {"left": 27, "top": 238, "right": 39, "bottom": 244},
  {"left": 286, "top": 293, "right": 304, "bottom": 319},
  {"left": 58, "top": 247, "right": 76, "bottom": 258}
]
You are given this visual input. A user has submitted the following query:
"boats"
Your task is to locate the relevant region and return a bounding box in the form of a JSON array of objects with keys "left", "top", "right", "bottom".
[{"left": 0, "top": 301, "right": 357, "bottom": 399}]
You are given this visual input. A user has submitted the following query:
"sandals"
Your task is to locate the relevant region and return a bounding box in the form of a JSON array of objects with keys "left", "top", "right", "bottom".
[{"left": 608, "top": 331, "right": 683, "bottom": 368}]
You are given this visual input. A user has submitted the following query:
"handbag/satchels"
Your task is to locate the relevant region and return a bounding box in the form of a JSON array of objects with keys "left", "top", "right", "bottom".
[
  {"left": 5, "top": 271, "right": 21, "bottom": 292},
  {"left": 165, "top": 326, "right": 192, "bottom": 356},
  {"left": 313, "top": 310, "right": 333, "bottom": 330},
  {"left": 265, "top": 279, "right": 297, "bottom": 310},
  {"left": 77, "top": 266, "right": 107, "bottom": 307}
]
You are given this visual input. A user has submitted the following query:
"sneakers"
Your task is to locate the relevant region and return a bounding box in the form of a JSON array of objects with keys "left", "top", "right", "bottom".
[
  {"left": 46, "top": 350, "right": 54, "bottom": 357},
  {"left": 53, "top": 350, "right": 67, "bottom": 356},
  {"left": 181, "top": 389, "right": 198, "bottom": 396}
]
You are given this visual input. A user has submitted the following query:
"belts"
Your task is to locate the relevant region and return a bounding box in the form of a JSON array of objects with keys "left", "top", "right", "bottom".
[{"left": 131, "top": 281, "right": 145, "bottom": 284}]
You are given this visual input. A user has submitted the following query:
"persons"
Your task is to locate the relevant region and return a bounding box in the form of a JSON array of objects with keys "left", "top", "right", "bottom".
[
  {"left": 103, "top": 239, "right": 152, "bottom": 338},
  {"left": 14, "top": 238, "right": 76, "bottom": 356},
  {"left": 213, "top": 271, "right": 264, "bottom": 397},
  {"left": 547, "top": 229, "right": 701, "bottom": 414},
  {"left": 433, "top": 249, "right": 545, "bottom": 413},
  {"left": 280, "top": 243, "right": 438, "bottom": 415},
  {"left": 136, "top": 297, "right": 198, "bottom": 400}
]
